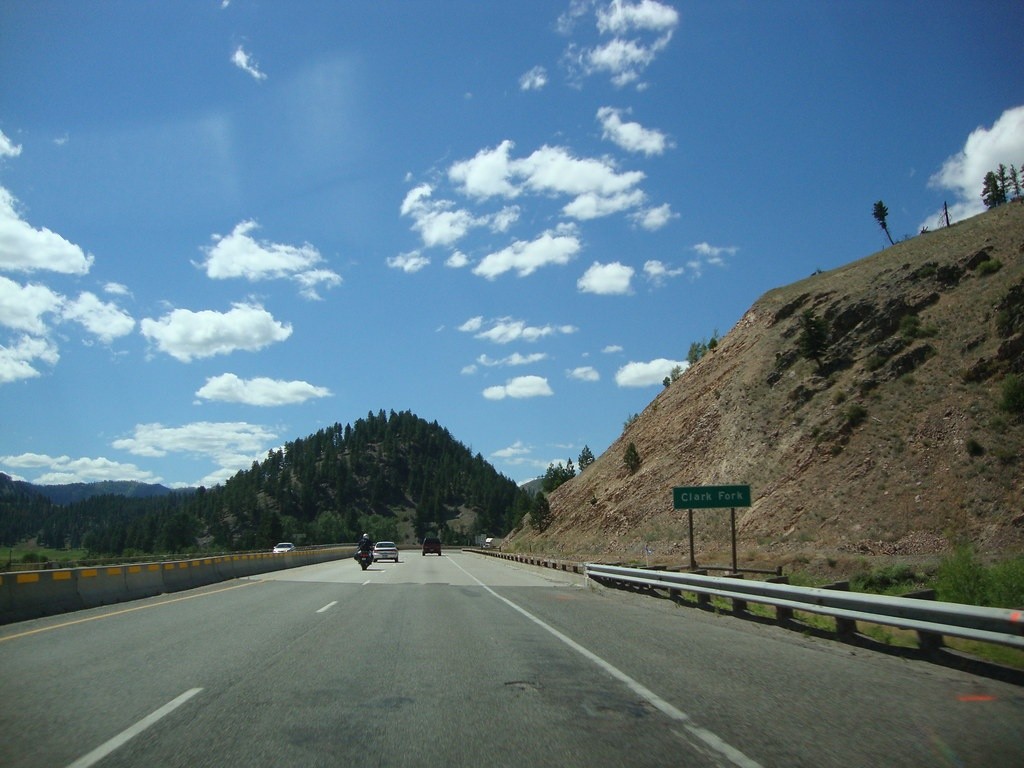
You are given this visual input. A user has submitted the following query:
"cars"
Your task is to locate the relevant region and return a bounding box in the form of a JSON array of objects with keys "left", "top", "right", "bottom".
[
  {"left": 373, "top": 541, "right": 400, "bottom": 563},
  {"left": 273, "top": 543, "right": 295, "bottom": 554}
]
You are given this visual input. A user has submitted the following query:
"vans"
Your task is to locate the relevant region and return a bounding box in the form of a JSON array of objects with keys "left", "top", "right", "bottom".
[{"left": 422, "top": 537, "right": 442, "bottom": 556}]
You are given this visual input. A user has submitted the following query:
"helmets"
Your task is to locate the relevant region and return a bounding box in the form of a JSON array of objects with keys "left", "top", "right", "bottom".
[{"left": 363, "top": 534, "right": 369, "bottom": 539}]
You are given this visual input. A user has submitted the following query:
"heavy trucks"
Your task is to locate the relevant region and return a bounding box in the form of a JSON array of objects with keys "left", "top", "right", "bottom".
[{"left": 485, "top": 537, "right": 505, "bottom": 548}]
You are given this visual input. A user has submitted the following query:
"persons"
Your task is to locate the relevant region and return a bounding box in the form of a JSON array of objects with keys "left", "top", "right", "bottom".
[{"left": 354, "top": 534, "right": 375, "bottom": 565}]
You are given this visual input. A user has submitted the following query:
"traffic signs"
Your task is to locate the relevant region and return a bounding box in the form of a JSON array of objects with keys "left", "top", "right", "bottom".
[{"left": 671, "top": 485, "right": 753, "bottom": 510}]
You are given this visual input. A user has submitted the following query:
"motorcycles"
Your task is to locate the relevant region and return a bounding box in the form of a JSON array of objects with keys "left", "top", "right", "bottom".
[{"left": 358, "top": 549, "right": 373, "bottom": 570}]
